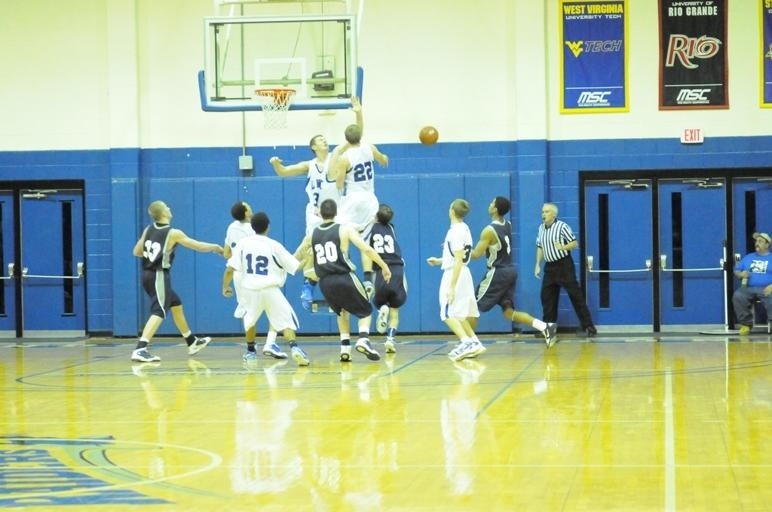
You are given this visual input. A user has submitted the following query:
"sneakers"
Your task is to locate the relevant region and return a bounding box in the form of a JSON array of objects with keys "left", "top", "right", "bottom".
[
  {"left": 262, "top": 343, "right": 288, "bottom": 358},
  {"left": 384, "top": 336, "right": 397, "bottom": 353},
  {"left": 242, "top": 349, "right": 257, "bottom": 362},
  {"left": 354, "top": 337, "right": 381, "bottom": 361},
  {"left": 541, "top": 322, "right": 558, "bottom": 350},
  {"left": 185, "top": 336, "right": 211, "bottom": 355},
  {"left": 300, "top": 279, "right": 378, "bottom": 313},
  {"left": 584, "top": 325, "right": 598, "bottom": 337},
  {"left": 290, "top": 345, "right": 311, "bottom": 366},
  {"left": 130, "top": 347, "right": 162, "bottom": 363},
  {"left": 447, "top": 339, "right": 487, "bottom": 360},
  {"left": 739, "top": 325, "right": 751, "bottom": 335},
  {"left": 375, "top": 305, "right": 390, "bottom": 335},
  {"left": 339, "top": 344, "right": 352, "bottom": 361}
]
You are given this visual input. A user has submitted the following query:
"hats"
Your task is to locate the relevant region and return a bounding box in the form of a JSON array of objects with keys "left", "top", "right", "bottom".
[{"left": 751, "top": 231, "right": 772, "bottom": 245}]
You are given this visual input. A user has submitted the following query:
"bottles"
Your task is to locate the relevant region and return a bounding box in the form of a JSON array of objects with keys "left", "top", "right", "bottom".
[{"left": 741, "top": 277, "right": 748, "bottom": 289}]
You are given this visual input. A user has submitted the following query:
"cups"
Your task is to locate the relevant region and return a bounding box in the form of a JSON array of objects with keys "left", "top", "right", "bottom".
[
  {"left": 661, "top": 255, "right": 666, "bottom": 268},
  {"left": 587, "top": 255, "right": 593, "bottom": 269},
  {"left": 735, "top": 253, "right": 740, "bottom": 264}
]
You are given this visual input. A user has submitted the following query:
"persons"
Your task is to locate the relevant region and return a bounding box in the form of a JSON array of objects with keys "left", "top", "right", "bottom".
[
  {"left": 292, "top": 198, "right": 393, "bottom": 362},
  {"left": 222, "top": 201, "right": 290, "bottom": 361},
  {"left": 221, "top": 209, "right": 311, "bottom": 368},
  {"left": 358, "top": 203, "right": 408, "bottom": 356},
  {"left": 335, "top": 123, "right": 390, "bottom": 304},
  {"left": 440, "top": 195, "right": 559, "bottom": 351},
  {"left": 130, "top": 199, "right": 225, "bottom": 363},
  {"left": 729, "top": 230, "right": 771, "bottom": 338},
  {"left": 425, "top": 197, "right": 487, "bottom": 361},
  {"left": 271, "top": 95, "right": 367, "bottom": 312},
  {"left": 534, "top": 200, "right": 598, "bottom": 338}
]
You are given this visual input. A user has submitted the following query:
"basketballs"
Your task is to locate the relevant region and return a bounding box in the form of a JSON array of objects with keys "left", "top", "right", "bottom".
[{"left": 419, "top": 126, "right": 438, "bottom": 146}]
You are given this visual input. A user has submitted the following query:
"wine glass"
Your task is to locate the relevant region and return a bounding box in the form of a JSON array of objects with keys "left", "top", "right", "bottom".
[{"left": 645, "top": 259, "right": 651, "bottom": 269}]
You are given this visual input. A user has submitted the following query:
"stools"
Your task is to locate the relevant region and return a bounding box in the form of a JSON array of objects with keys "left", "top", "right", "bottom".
[{"left": 748, "top": 297, "right": 772, "bottom": 334}]
